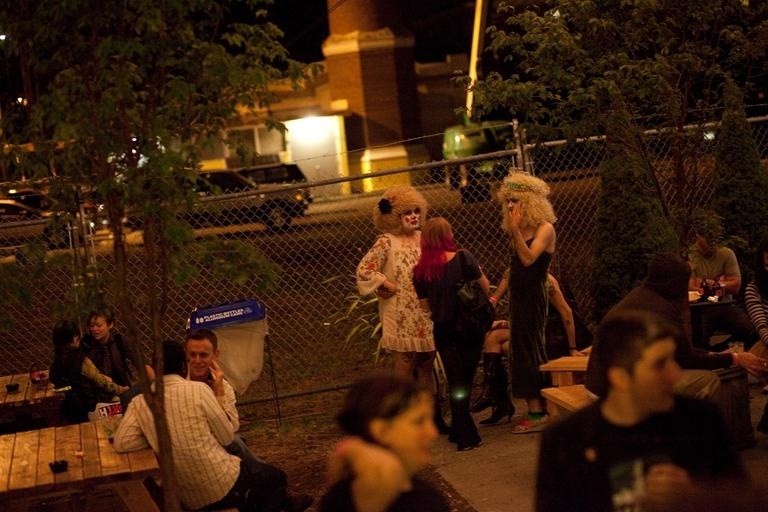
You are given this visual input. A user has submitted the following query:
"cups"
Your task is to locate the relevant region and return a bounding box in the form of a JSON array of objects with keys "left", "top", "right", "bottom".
[
  {"left": 40, "top": 377, "right": 49, "bottom": 388},
  {"left": 718, "top": 285, "right": 725, "bottom": 298}
]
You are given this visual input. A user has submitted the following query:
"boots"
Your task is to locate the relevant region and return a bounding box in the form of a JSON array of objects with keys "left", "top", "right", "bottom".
[{"left": 470, "top": 352, "right": 516, "bottom": 425}]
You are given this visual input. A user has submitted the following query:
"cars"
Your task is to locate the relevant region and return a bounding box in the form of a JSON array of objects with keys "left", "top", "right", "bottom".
[{"left": 236, "top": 164, "right": 313, "bottom": 203}]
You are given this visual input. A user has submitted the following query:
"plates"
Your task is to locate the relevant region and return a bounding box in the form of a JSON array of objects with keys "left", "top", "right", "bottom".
[{"left": 693, "top": 331, "right": 732, "bottom": 354}]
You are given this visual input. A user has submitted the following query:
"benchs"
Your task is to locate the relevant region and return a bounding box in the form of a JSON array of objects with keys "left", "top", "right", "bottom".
[
  {"left": 540, "top": 357, "right": 591, "bottom": 387},
  {"left": 540, "top": 384, "right": 599, "bottom": 420}
]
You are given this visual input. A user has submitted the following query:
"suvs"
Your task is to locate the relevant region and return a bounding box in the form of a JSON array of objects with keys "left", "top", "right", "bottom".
[
  {"left": 119, "top": 168, "right": 311, "bottom": 245},
  {"left": 0, "top": 163, "right": 102, "bottom": 248}
]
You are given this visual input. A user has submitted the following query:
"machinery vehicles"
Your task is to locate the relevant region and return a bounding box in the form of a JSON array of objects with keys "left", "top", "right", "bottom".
[{"left": 441, "top": 0, "right": 517, "bottom": 189}]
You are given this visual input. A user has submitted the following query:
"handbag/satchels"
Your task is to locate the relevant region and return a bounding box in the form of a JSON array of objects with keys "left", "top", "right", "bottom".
[{"left": 457, "top": 280, "right": 496, "bottom": 318}]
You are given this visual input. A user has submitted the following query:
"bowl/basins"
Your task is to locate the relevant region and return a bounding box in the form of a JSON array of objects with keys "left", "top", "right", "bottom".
[
  {"left": 5, "top": 383, "right": 19, "bottom": 394},
  {"left": 48, "top": 460, "right": 67, "bottom": 474}
]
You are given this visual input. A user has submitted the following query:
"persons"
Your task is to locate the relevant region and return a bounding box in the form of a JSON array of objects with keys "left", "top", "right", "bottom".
[
  {"left": 315, "top": 375, "right": 453, "bottom": 512},
  {"left": 357, "top": 184, "right": 444, "bottom": 428},
  {"left": 584, "top": 254, "right": 768, "bottom": 405},
  {"left": 184, "top": 328, "right": 255, "bottom": 463},
  {"left": 471, "top": 266, "right": 592, "bottom": 425},
  {"left": 81, "top": 305, "right": 154, "bottom": 390},
  {"left": 413, "top": 216, "right": 490, "bottom": 452},
  {"left": 495, "top": 167, "right": 558, "bottom": 433},
  {"left": 49, "top": 320, "right": 130, "bottom": 425},
  {"left": 742, "top": 234, "right": 768, "bottom": 433},
  {"left": 535, "top": 307, "right": 751, "bottom": 511},
  {"left": 115, "top": 341, "right": 289, "bottom": 511},
  {"left": 687, "top": 229, "right": 760, "bottom": 352}
]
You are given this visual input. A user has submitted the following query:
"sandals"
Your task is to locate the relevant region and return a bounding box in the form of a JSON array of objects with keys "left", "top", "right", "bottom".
[{"left": 509, "top": 411, "right": 549, "bottom": 432}]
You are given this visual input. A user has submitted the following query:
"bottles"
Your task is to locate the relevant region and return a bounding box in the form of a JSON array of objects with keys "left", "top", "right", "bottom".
[
  {"left": 699, "top": 277, "right": 708, "bottom": 300},
  {"left": 29, "top": 363, "right": 39, "bottom": 384},
  {"left": 711, "top": 278, "right": 722, "bottom": 301}
]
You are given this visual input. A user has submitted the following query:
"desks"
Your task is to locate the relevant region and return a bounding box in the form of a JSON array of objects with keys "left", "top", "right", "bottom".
[
  {"left": 0, "top": 419, "right": 162, "bottom": 512},
  {"left": 0, "top": 370, "right": 66, "bottom": 427}
]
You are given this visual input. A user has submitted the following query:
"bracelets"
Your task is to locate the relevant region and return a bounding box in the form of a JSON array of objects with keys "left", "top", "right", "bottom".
[
  {"left": 731, "top": 352, "right": 737, "bottom": 368},
  {"left": 567, "top": 346, "right": 577, "bottom": 350},
  {"left": 489, "top": 296, "right": 497, "bottom": 303}
]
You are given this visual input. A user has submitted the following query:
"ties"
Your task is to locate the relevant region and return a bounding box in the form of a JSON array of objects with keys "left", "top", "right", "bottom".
[{"left": 101, "top": 346, "right": 113, "bottom": 378}]
